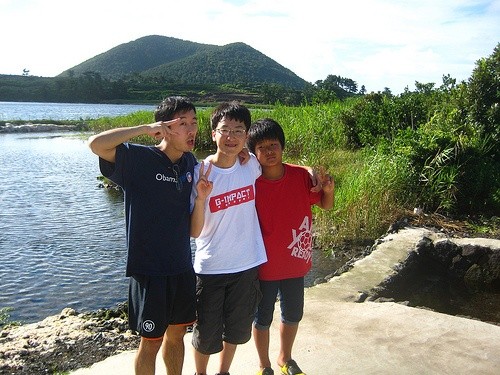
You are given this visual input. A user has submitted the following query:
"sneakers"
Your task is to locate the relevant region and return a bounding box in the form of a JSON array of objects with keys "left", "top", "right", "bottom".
[
  {"left": 256, "top": 367, "right": 274, "bottom": 375},
  {"left": 277, "top": 357, "right": 307, "bottom": 375}
]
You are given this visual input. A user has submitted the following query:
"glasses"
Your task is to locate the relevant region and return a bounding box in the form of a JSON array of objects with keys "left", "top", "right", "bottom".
[{"left": 215, "top": 126, "right": 249, "bottom": 137}]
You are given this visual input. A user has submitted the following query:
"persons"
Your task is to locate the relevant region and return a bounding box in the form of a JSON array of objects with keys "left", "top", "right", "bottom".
[
  {"left": 89, "top": 95, "right": 249, "bottom": 375},
  {"left": 189, "top": 102, "right": 321, "bottom": 375},
  {"left": 246, "top": 115, "right": 335, "bottom": 375}
]
